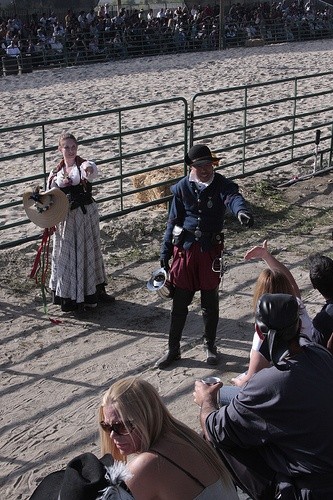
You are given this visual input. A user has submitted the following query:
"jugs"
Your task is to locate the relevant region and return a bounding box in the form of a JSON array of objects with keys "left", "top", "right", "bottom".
[{"left": 147, "top": 267, "right": 175, "bottom": 301}]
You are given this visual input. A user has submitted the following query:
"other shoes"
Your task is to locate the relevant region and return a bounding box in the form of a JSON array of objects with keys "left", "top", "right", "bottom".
[{"left": 96, "top": 289, "right": 115, "bottom": 303}]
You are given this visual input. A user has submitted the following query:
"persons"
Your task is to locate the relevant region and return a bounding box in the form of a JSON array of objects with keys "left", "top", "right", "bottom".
[
  {"left": 155, "top": 144, "right": 254, "bottom": 369},
  {"left": 96, "top": 376, "right": 240, "bottom": 500},
  {"left": 193, "top": 240, "right": 333, "bottom": 500},
  {"left": 45, "top": 134, "right": 115, "bottom": 312},
  {"left": 0, "top": 0, "right": 333, "bottom": 78}
]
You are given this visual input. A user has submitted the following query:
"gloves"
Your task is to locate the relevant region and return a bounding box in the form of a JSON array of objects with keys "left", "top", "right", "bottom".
[
  {"left": 160, "top": 260, "right": 171, "bottom": 273},
  {"left": 238, "top": 210, "right": 254, "bottom": 229}
]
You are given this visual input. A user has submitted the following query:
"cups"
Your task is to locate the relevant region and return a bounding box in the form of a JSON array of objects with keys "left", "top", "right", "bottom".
[{"left": 201, "top": 377, "right": 222, "bottom": 409}]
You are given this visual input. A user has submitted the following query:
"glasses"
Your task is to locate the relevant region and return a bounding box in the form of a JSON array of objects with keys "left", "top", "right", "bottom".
[{"left": 99, "top": 418, "right": 136, "bottom": 436}]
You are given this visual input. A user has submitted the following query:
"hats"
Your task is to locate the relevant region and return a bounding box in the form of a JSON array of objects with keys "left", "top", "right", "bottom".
[
  {"left": 28, "top": 452, "right": 114, "bottom": 500},
  {"left": 184, "top": 145, "right": 223, "bottom": 167},
  {"left": 22, "top": 186, "right": 70, "bottom": 227}
]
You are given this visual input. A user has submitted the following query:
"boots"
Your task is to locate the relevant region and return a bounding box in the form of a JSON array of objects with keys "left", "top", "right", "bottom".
[
  {"left": 155, "top": 309, "right": 187, "bottom": 367},
  {"left": 202, "top": 308, "right": 219, "bottom": 364}
]
